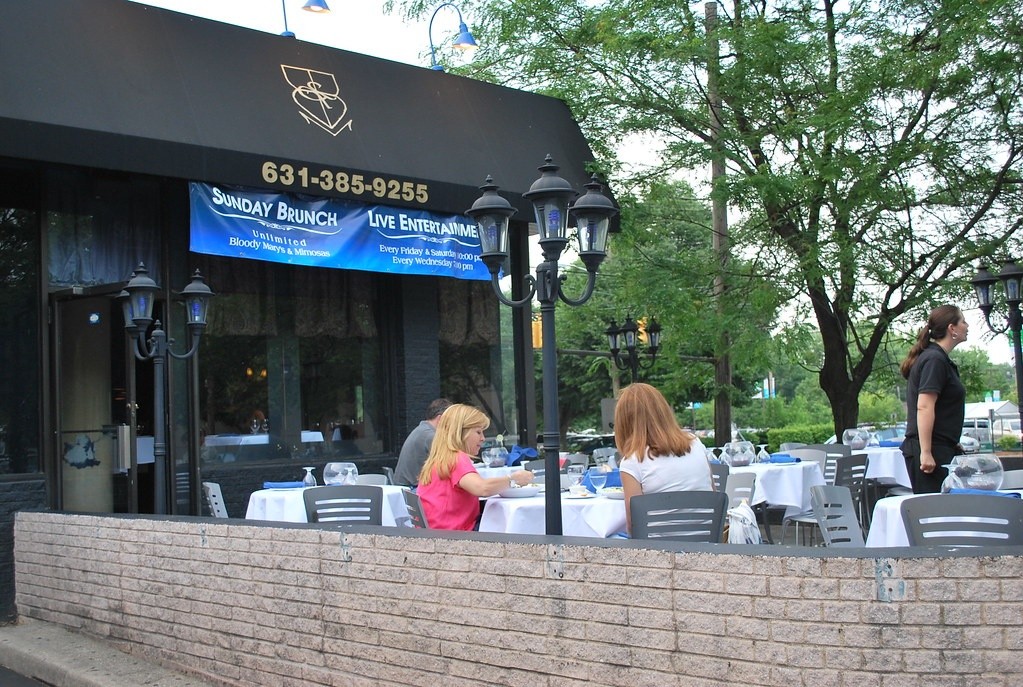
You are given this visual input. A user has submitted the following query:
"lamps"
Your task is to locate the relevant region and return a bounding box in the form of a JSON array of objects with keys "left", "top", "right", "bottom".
[
  {"left": 280, "top": 0, "right": 331, "bottom": 38},
  {"left": 429, "top": 3, "right": 479, "bottom": 70}
]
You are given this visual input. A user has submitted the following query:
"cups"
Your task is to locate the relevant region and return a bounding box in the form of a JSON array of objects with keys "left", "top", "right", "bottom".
[
  {"left": 567, "top": 466, "right": 584, "bottom": 485},
  {"left": 569, "top": 486, "right": 586, "bottom": 496}
]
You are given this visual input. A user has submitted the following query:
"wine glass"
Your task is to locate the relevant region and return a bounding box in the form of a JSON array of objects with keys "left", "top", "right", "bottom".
[
  {"left": 942, "top": 464, "right": 963, "bottom": 494},
  {"left": 852, "top": 432, "right": 862, "bottom": 443},
  {"left": 867, "top": 432, "right": 879, "bottom": 447},
  {"left": 334, "top": 416, "right": 364, "bottom": 427},
  {"left": 734, "top": 446, "right": 753, "bottom": 464},
  {"left": 482, "top": 451, "right": 493, "bottom": 470},
  {"left": 345, "top": 468, "right": 355, "bottom": 484},
  {"left": 706, "top": 448, "right": 720, "bottom": 464},
  {"left": 718, "top": 447, "right": 731, "bottom": 467},
  {"left": 249, "top": 418, "right": 270, "bottom": 434},
  {"left": 756, "top": 444, "right": 771, "bottom": 464},
  {"left": 301, "top": 467, "right": 317, "bottom": 489},
  {"left": 492, "top": 439, "right": 503, "bottom": 460},
  {"left": 589, "top": 467, "right": 607, "bottom": 500}
]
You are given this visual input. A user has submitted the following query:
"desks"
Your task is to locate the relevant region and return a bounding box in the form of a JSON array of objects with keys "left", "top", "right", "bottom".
[
  {"left": 865, "top": 494, "right": 946, "bottom": 546},
  {"left": 846, "top": 447, "right": 911, "bottom": 529},
  {"left": 204, "top": 430, "right": 325, "bottom": 462},
  {"left": 730, "top": 461, "right": 824, "bottom": 544},
  {"left": 474, "top": 463, "right": 532, "bottom": 481},
  {"left": 478, "top": 482, "right": 627, "bottom": 538},
  {"left": 244, "top": 485, "right": 410, "bottom": 527}
]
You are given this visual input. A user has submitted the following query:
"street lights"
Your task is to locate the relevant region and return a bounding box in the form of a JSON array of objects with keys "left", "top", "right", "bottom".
[
  {"left": 603, "top": 312, "right": 664, "bottom": 383},
  {"left": 465, "top": 152, "right": 619, "bottom": 531},
  {"left": 115, "top": 261, "right": 216, "bottom": 514},
  {"left": 965, "top": 252, "right": 1023, "bottom": 435}
]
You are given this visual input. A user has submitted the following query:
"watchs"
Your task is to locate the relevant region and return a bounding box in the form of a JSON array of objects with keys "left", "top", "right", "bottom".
[{"left": 506, "top": 473, "right": 516, "bottom": 489}]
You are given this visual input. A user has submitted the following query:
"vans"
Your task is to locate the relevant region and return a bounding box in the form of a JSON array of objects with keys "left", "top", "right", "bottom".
[
  {"left": 962, "top": 419, "right": 988, "bottom": 443},
  {"left": 989, "top": 418, "right": 1023, "bottom": 442}
]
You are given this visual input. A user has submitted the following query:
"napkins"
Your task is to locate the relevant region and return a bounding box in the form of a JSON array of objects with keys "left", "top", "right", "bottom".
[
  {"left": 769, "top": 454, "right": 801, "bottom": 464},
  {"left": 264, "top": 481, "right": 303, "bottom": 488},
  {"left": 879, "top": 441, "right": 903, "bottom": 447},
  {"left": 505, "top": 446, "right": 538, "bottom": 465},
  {"left": 948, "top": 489, "right": 1021, "bottom": 500},
  {"left": 581, "top": 469, "right": 621, "bottom": 493}
]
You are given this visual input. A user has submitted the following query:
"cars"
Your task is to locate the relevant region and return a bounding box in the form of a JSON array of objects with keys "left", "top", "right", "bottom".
[
  {"left": 558, "top": 434, "right": 616, "bottom": 473},
  {"left": 857, "top": 426, "right": 909, "bottom": 443},
  {"left": 957, "top": 435, "right": 981, "bottom": 452}
]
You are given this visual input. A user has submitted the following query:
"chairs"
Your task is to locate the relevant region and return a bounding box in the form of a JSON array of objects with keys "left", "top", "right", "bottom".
[{"left": 201, "top": 426, "right": 1023, "bottom": 555}]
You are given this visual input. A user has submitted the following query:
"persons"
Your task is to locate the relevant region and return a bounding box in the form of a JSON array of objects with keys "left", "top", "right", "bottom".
[
  {"left": 898, "top": 304, "right": 969, "bottom": 495},
  {"left": 414, "top": 403, "right": 534, "bottom": 532},
  {"left": 605, "top": 382, "right": 716, "bottom": 543},
  {"left": 393, "top": 398, "right": 454, "bottom": 493}
]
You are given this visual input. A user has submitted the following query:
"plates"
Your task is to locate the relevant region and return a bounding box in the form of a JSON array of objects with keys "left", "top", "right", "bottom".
[
  {"left": 601, "top": 486, "right": 625, "bottom": 499},
  {"left": 499, "top": 487, "right": 544, "bottom": 498}
]
